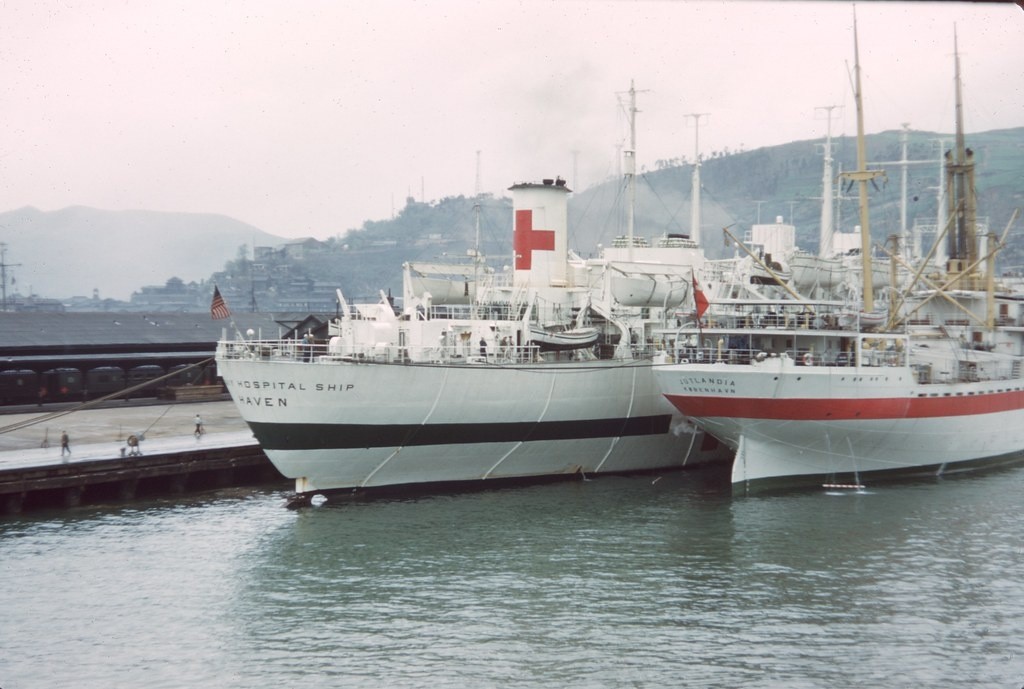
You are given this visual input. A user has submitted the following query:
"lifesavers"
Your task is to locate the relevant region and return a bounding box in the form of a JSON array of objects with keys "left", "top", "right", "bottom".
[{"left": 803, "top": 352, "right": 815, "bottom": 366}]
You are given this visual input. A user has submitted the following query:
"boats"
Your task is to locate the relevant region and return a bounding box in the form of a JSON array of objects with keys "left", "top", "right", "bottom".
[
  {"left": 654, "top": 0, "right": 1023, "bottom": 497},
  {"left": 214, "top": 69, "right": 730, "bottom": 506}
]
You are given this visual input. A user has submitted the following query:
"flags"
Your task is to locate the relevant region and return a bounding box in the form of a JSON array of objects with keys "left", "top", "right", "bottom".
[
  {"left": 211, "top": 286, "right": 228, "bottom": 319},
  {"left": 692, "top": 276, "right": 709, "bottom": 319}
]
